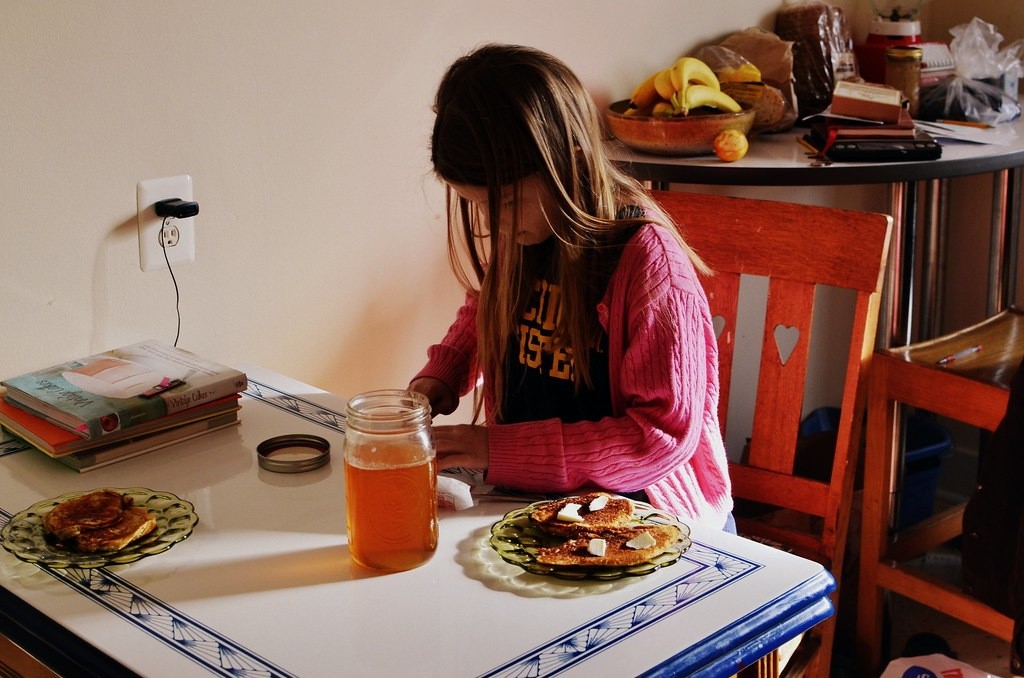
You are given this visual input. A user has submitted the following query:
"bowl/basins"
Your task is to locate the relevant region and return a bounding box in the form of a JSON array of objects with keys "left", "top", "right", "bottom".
[{"left": 605, "top": 100, "right": 755, "bottom": 155}]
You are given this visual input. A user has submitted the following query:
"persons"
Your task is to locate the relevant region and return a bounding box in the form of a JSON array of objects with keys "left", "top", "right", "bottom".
[{"left": 402, "top": 43, "right": 736, "bottom": 534}]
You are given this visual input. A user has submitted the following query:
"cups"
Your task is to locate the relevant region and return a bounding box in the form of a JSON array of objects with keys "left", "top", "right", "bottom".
[{"left": 342, "top": 390, "right": 437, "bottom": 571}]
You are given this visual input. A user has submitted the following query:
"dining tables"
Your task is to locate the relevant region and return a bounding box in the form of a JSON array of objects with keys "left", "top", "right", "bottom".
[{"left": 0, "top": 361, "right": 840, "bottom": 678}]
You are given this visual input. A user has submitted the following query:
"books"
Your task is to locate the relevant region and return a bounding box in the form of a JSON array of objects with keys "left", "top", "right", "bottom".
[{"left": 0, "top": 339, "right": 247, "bottom": 473}]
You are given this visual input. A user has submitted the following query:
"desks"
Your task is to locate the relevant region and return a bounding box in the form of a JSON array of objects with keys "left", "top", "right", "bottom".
[{"left": 595, "top": 112, "right": 1024, "bottom": 568}]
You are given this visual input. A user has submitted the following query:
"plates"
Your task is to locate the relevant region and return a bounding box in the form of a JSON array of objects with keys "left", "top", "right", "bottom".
[
  {"left": 489, "top": 493, "right": 691, "bottom": 581},
  {"left": 2, "top": 487, "right": 198, "bottom": 570}
]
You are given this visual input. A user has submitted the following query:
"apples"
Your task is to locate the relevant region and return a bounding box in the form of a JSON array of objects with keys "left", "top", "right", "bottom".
[{"left": 712, "top": 131, "right": 748, "bottom": 162}]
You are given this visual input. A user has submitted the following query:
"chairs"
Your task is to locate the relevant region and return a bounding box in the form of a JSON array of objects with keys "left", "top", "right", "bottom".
[
  {"left": 851, "top": 352, "right": 1017, "bottom": 678},
  {"left": 643, "top": 187, "right": 895, "bottom": 678}
]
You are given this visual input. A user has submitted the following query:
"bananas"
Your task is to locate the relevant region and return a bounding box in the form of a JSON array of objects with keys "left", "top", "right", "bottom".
[{"left": 621, "top": 57, "right": 741, "bottom": 118}]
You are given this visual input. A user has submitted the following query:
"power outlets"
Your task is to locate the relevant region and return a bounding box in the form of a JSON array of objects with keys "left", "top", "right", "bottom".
[{"left": 136, "top": 173, "right": 196, "bottom": 272}]
